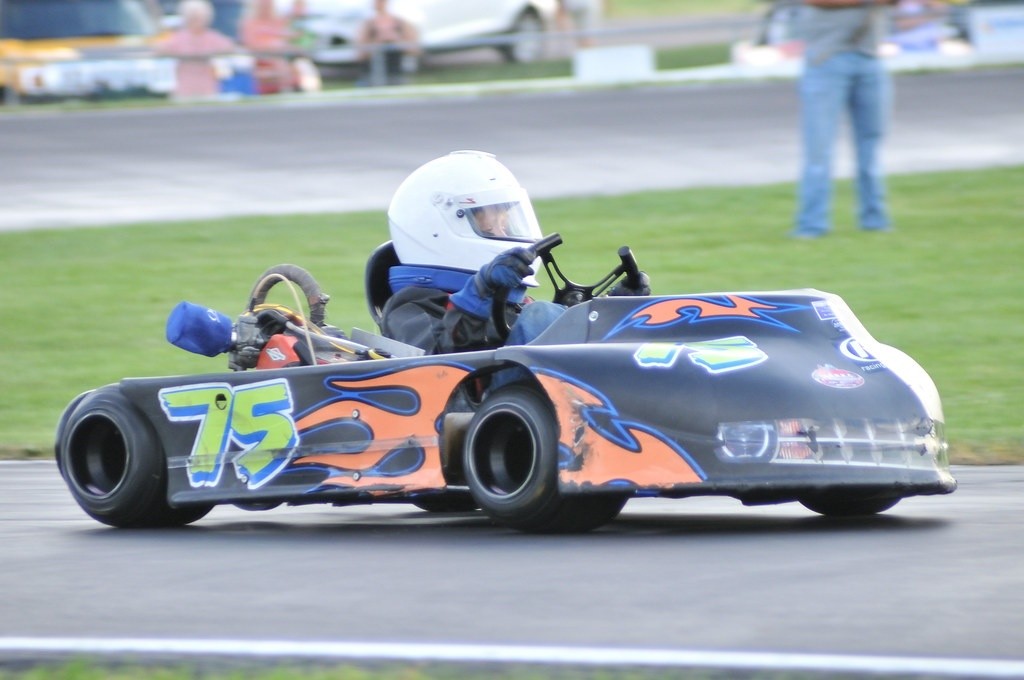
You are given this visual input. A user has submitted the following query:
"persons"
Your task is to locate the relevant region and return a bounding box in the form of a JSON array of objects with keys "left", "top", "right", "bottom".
[
  {"left": 786, "top": 0, "right": 896, "bottom": 240},
  {"left": 151, "top": 0, "right": 257, "bottom": 96},
  {"left": 359, "top": 0, "right": 422, "bottom": 74},
  {"left": 380, "top": 150, "right": 650, "bottom": 359},
  {"left": 239, "top": 0, "right": 322, "bottom": 90}
]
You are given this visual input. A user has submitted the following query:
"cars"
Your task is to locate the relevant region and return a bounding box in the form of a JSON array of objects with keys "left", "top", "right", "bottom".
[
  {"left": 0, "top": 0, "right": 250, "bottom": 104},
  {"left": 272, "top": 0, "right": 558, "bottom": 73},
  {"left": 767, "top": 4, "right": 972, "bottom": 67}
]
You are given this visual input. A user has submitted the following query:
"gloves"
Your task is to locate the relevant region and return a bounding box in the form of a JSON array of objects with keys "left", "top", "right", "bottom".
[
  {"left": 449, "top": 245, "right": 537, "bottom": 321},
  {"left": 607, "top": 270, "right": 650, "bottom": 296}
]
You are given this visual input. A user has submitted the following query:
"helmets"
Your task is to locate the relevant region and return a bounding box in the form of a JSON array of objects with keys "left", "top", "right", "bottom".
[{"left": 388, "top": 151, "right": 546, "bottom": 286}]
